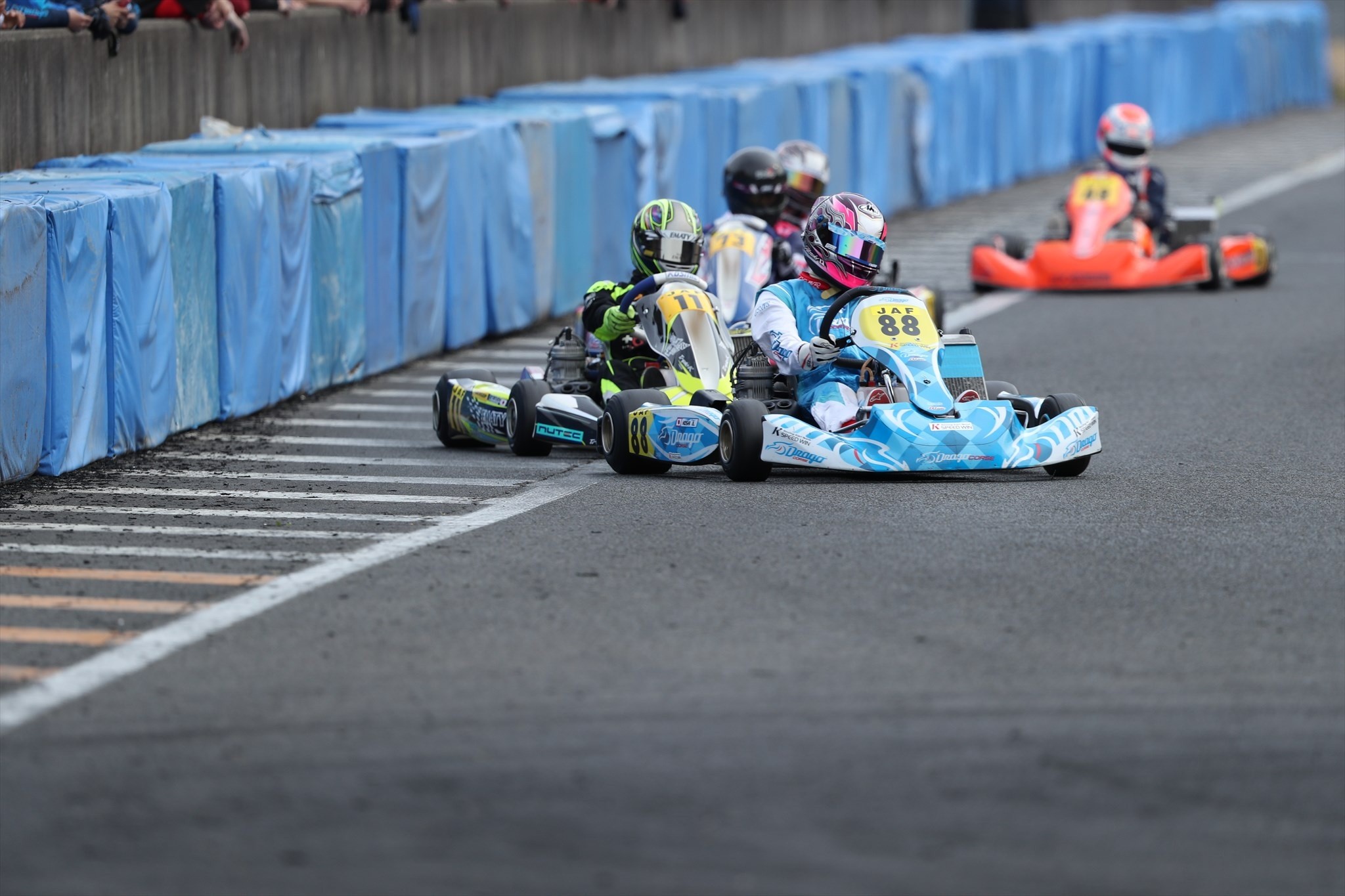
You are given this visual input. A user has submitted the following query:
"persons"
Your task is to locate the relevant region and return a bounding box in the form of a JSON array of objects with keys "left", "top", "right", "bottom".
[
  {"left": 1059, "top": 103, "right": 1178, "bottom": 257},
  {"left": 582, "top": 199, "right": 706, "bottom": 408},
  {"left": 702, "top": 139, "right": 830, "bottom": 288},
  {"left": 751, "top": 192, "right": 887, "bottom": 430},
  {"left": 0, "top": 0, "right": 428, "bottom": 53}
]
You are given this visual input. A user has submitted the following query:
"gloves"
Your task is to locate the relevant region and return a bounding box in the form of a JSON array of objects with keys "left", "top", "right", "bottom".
[
  {"left": 799, "top": 333, "right": 841, "bottom": 371},
  {"left": 773, "top": 236, "right": 795, "bottom": 267},
  {"left": 606, "top": 305, "right": 637, "bottom": 334}
]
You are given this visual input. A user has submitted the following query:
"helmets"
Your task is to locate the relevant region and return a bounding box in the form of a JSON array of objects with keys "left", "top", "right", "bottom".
[
  {"left": 1097, "top": 101, "right": 1153, "bottom": 172},
  {"left": 723, "top": 144, "right": 789, "bottom": 225},
  {"left": 803, "top": 191, "right": 887, "bottom": 290},
  {"left": 774, "top": 138, "right": 831, "bottom": 221},
  {"left": 630, "top": 199, "right": 705, "bottom": 282}
]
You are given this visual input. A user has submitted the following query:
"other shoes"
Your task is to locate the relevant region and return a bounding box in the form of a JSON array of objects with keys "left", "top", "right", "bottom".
[
  {"left": 863, "top": 389, "right": 891, "bottom": 420},
  {"left": 954, "top": 390, "right": 981, "bottom": 403}
]
